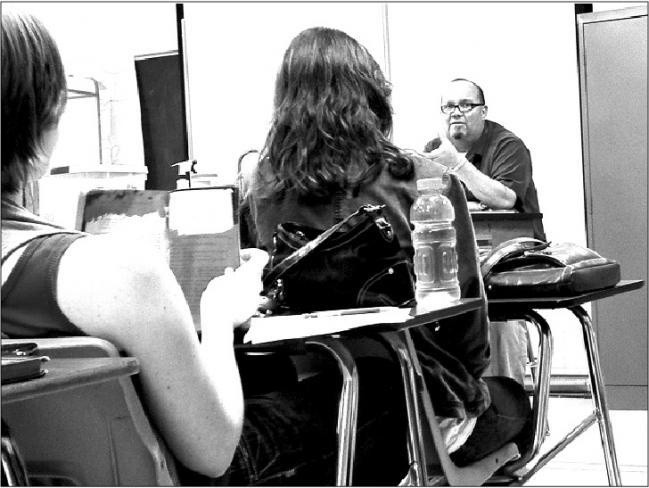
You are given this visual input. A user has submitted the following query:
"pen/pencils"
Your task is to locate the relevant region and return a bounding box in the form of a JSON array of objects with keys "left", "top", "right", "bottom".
[{"left": 332, "top": 309, "right": 380, "bottom": 316}]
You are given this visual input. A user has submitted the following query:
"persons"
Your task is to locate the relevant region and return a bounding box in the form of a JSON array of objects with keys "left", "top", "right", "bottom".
[
  {"left": 241, "top": 24, "right": 536, "bottom": 467},
  {"left": 415, "top": 76, "right": 551, "bottom": 389},
  {"left": 0, "top": 11, "right": 410, "bottom": 485}
]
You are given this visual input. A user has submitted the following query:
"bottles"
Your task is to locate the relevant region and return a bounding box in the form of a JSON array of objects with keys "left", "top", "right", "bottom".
[{"left": 407, "top": 178, "right": 463, "bottom": 304}]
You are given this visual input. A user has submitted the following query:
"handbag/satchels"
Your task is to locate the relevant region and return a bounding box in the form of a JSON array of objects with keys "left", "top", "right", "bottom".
[
  {"left": 272, "top": 215, "right": 418, "bottom": 308},
  {"left": 484, "top": 243, "right": 621, "bottom": 298}
]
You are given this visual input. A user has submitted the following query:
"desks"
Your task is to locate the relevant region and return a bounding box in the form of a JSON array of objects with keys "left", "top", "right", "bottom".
[{"left": 470, "top": 212, "right": 543, "bottom": 261}]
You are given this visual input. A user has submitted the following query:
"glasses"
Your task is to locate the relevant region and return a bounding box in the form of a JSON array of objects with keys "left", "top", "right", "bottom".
[{"left": 439, "top": 101, "right": 485, "bottom": 114}]
